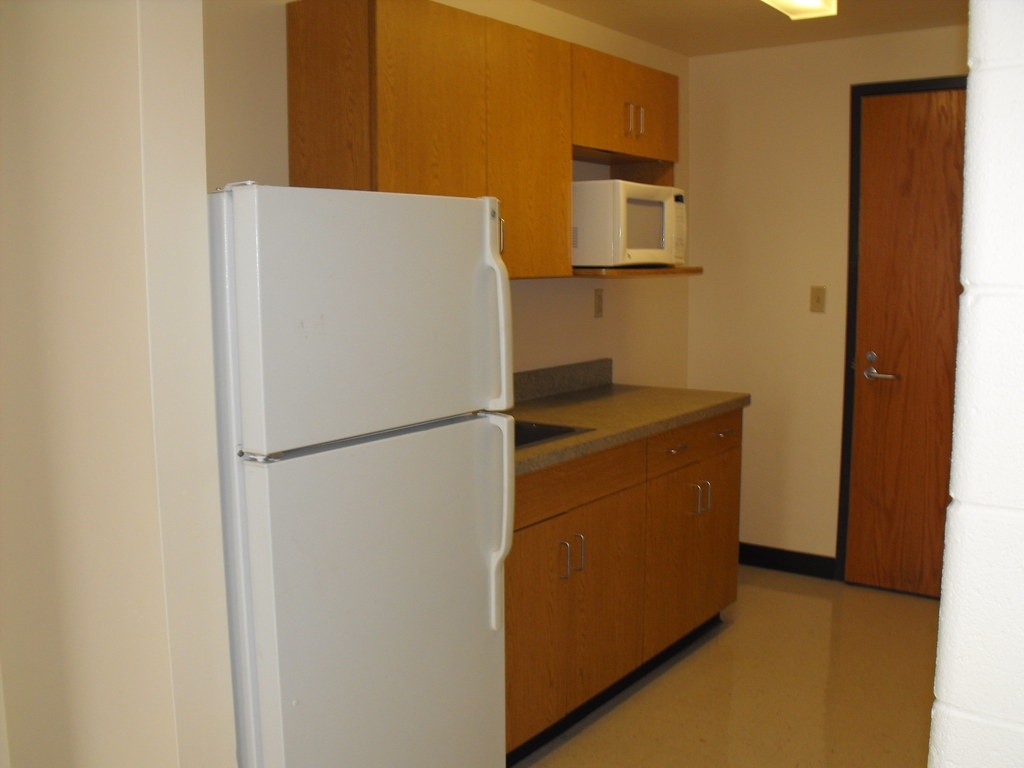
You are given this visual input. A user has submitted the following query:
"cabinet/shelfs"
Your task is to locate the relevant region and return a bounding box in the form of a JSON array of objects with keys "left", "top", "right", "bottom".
[
  {"left": 505, "top": 409, "right": 745, "bottom": 768},
  {"left": 285, "top": 0, "right": 704, "bottom": 278}
]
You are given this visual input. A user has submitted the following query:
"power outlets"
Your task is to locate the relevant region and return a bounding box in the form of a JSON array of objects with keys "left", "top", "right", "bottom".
[{"left": 594, "top": 288, "right": 604, "bottom": 317}]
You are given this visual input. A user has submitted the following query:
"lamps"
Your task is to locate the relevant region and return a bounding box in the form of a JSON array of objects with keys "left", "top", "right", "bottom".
[{"left": 760, "top": 0, "right": 838, "bottom": 21}]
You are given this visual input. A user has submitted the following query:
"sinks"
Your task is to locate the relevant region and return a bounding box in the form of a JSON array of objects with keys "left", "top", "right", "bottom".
[{"left": 514, "top": 419, "right": 596, "bottom": 449}]
